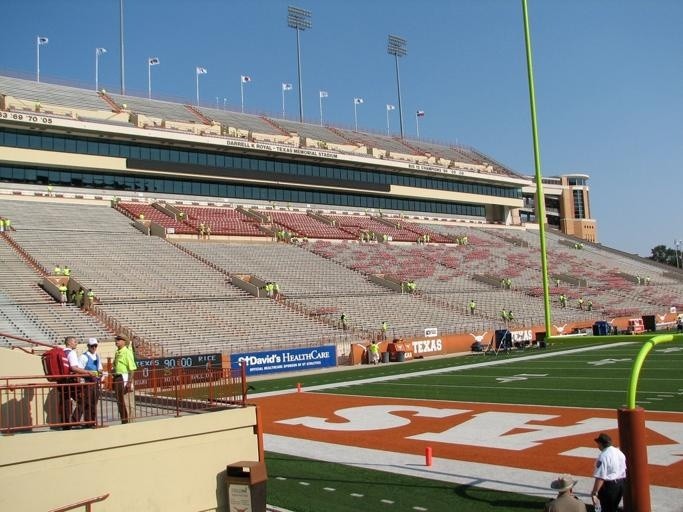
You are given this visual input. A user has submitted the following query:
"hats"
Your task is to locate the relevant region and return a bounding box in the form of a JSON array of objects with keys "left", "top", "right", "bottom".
[
  {"left": 115, "top": 333, "right": 130, "bottom": 344},
  {"left": 89, "top": 338, "right": 99, "bottom": 345},
  {"left": 550, "top": 474, "right": 578, "bottom": 492},
  {"left": 594, "top": 433, "right": 611, "bottom": 444}
]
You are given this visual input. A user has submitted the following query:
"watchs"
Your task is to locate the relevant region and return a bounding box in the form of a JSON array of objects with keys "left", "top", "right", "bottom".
[{"left": 591, "top": 491, "right": 598, "bottom": 496}]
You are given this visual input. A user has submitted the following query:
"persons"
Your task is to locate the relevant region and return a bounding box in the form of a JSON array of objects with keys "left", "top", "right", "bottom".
[
  {"left": 77, "top": 337, "right": 104, "bottom": 429},
  {"left": 590, "top": 432, "right": 628, "bottom": 512},
  {"left": 274, "top": 228, "right": 309, "bottom": 245},
  {"left": 54, "top": 264, "right": 95, "bottom": 315},
  {"left": 209, "top": 117, "right": 329, "bottom": 149},
  {"left": 34, "top": 98, "right": 43, "bottom": 112},
  {"left": 272, "top": 201, "right": 293, "bottom": 210},
  {"left": 634, "top": 274, "right": 650, "bottom": 286},
  {"left": 455, "top": 233, "right": 468, "bottom": 245},
  {"left": 56, "top": 336, "right": 96, "bottom": 430},
  {"left": 333, "top": 209, "right": 431, "bottom": 245},
  {"left": 676, "top": 317, "right": 682, "bottom": 333},
  {"left": 110, "top": 333, "right": 138, "bottom": 424},
  {"left": 112, "top": 196, "right": 212, "bottom": 240},
  {"left": 400, "top": 279, "right": 417, "bottom": 294},
  {"left": 47, "top": 183, "right": 53, "bottom": 197},
  {"left": 469, "top": 277, "right": 515, "bottom": 322},
  {"left": 542, "top": 472, "right": 588, "bottom": 512},
  {"left": 340, "top": 312, "right": 387, "bottom": 363},
  {"left": 574, "top": 240, "right": 584, "bottom": 250},
  {"left": 556, "top": 277, "right": 592, "bottom": 311},
  {"left": 265, "top": 281, "right": 279, "bottom": 301},
  {"left": 0, "top": 217, "right": 12, "bottom": 232}
]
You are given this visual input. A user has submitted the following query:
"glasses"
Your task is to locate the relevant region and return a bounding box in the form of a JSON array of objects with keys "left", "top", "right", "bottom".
[{"left": 91, "top": 345, "right": 97, "bottom": 347}]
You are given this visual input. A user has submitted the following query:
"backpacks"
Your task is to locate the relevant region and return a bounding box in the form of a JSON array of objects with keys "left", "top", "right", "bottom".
[{"left": 41, "top": 347, "right": 70, "bottom": 382}]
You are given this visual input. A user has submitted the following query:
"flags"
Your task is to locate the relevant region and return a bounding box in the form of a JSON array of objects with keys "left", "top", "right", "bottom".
[
  {"left": 197, "top": 67, "right": 208, "bottom": 74},
  {"left": 283, "top": 83, "right": 293, "bottom": 90},
  {"left": 149, "top": 57, "right": 160, "bottom": 65},
  {"left": 38, "top": 36, "right": 48, "bottom": 45},
  {"left": 96, "top": 48, "right": 107, "bottom": 55},
  {"left": 387, "top": 104, "right": 395, "bottom": 111},
  {"left": 355, "top": 98, "right": 363, "bottom": 104},
  {"left": 417, "top": 110, "right": 424, "bottom": 118},
  {"left": 242, "top": 76, "right": 251, "bottom": 83},
  {"left": 320, "top": 92, "right": 328, "bottom": 97}
]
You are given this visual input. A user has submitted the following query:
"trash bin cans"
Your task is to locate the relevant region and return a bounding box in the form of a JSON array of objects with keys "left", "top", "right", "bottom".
[
  {"left": 382, "top": 352, "right": 389, "bottom": 363},
  {"left": 593, "top": 321, "right": 608, "bottom": 336},
  {"left": 397, "top": 351, "right": 405, "bottom": 361},
  {"left": 535, "top": 332, "right": 546, "bottom": 344},
  {"left": 223, "top": 460, "right": 268, "bottom": 512},
  {"left": 642, "top": 315, "right": 656, "bottom": 331}
]
